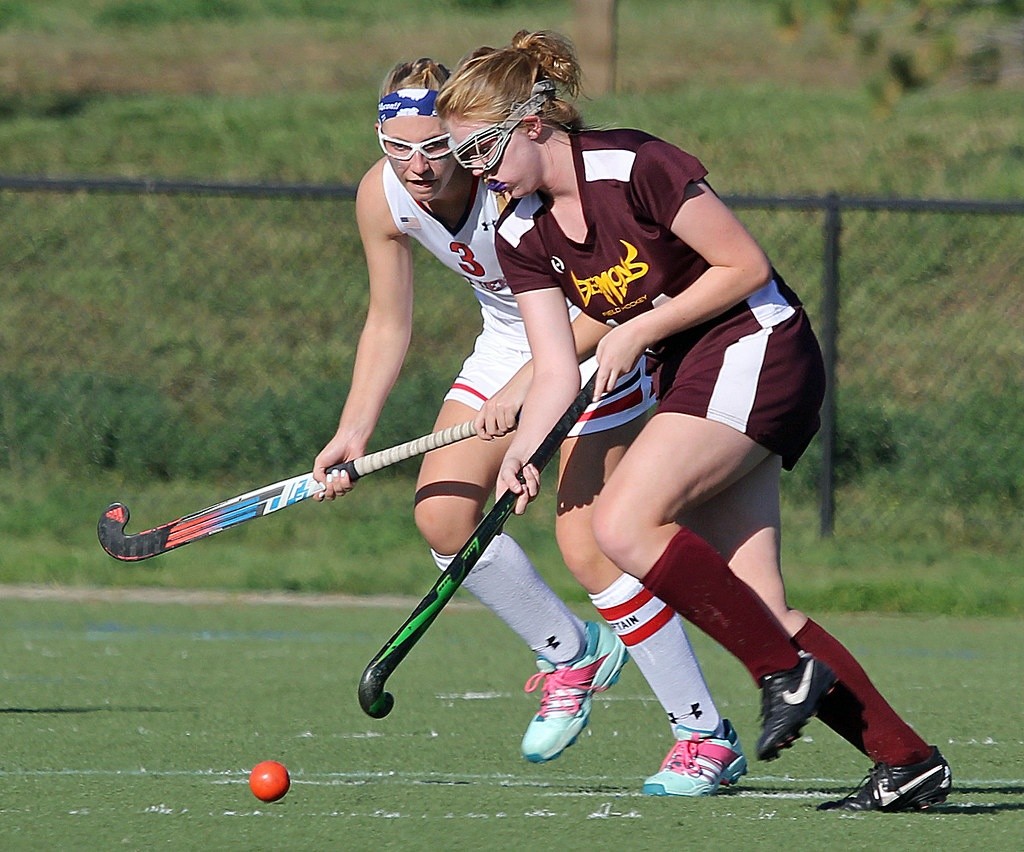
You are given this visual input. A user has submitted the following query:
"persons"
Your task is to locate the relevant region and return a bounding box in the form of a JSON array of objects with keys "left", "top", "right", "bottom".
[
  {"left": 435, "top": 27, "right": 953, "bottom": 813},
  {"left": 311, "top": 56, "right": 747, "bottom": 796}
]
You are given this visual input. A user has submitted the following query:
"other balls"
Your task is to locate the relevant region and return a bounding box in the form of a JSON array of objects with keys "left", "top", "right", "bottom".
[{"left": 248, "top": 761, "right": 290, "bottom": 802}]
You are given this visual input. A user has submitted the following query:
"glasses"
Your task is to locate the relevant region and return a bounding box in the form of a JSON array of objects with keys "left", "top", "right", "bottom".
[
  {"left": 447, "top": 124, "right": 511, "bottom": 171},
  {"left": 377, "top": 118, "right": 451, "bottom": 161}
]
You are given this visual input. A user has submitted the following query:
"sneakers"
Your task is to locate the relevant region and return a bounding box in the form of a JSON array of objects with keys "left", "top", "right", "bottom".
[
  {"left": 520, "top": 621, "right": 629, "bottom": 764},
  {"left": 817, "top": 745, "right": 952, "bottom": 814},
  {"left": 640, "top": 718, "right": 748, "bottom": 798},
  {"left": 754, "top": 649, "right": 839, "bottom": 762}
]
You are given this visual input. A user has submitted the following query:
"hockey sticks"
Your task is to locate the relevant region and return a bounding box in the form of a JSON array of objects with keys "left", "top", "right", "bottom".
[
  {"left": 358, "top": 367, "right": 607, "bottom": 719},
  {"left": 95, "top": 406, "right": 521, "bottom": 563}
]
